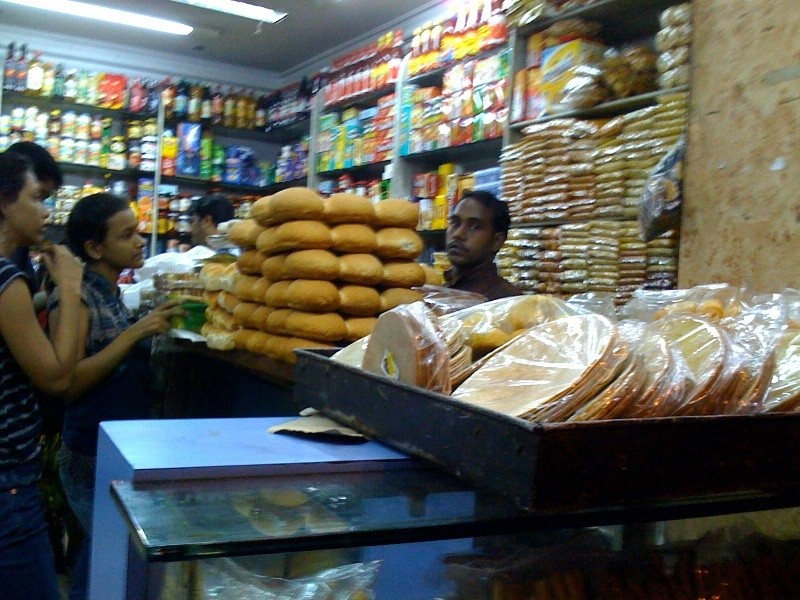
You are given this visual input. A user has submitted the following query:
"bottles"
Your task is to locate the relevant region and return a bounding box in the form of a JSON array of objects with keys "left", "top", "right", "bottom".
[
  {"left": 63, "top": 71, "right": 78, "bottom": 103},
  {"left": 236, "top": 87, "right": 247, "bottom": 129},
  {"left": 174, "top": 79, "right": 191, "bottom": 118},
  {"left": 41, "top": 62, "right": 55, "bottom": 98},
  {"left": 2, "top": 42, "right": 17, "bottom": 91},
  {"left": 223, "top": 85, "right": 238, "bottom": 127},
  {"left": 25, "top": 49, "right": 44, "bottom": 96},
  {"left": 440, "top": 0, "right": 492, "bottom": 65},
  {"left": 15, "top": 44, "right": 30, "bottom": 91},
  {"left": 163, "top": 77, "right": 176, "bottom": 119},
  {"left": 256, "top": 92, "right": 266, "bottom": 131},
  {"left": 189, "top": 81, "right": 203, "bottom": 122},
  {"left": 408, "top": 20, "right": 421, "bottom": 76},
  {"left": 296, "top": 72, "right": 320, "bottom": 122},
  {"left": 53, "top": 63, "right": 65, "bottom": 100},
  {"left": 211, "top": 83, "right": 224, "bottom": 127},
  {"left": 320, "top": 47, "right": 353, "bottom": 104},
  {"left": 419, "top": 22, "right": 432, "bottom": 72},
  {"left": 201, "top": 83, "right": 213, "bottom": 126},
  {"left": 353, "top": 44, "right": 379, "bottom": 94},
  {"left": 378, "top": 30, "right": 403, "bottom": 84},
  {"left": 246, "top": 89, "right": 257, "bottom": 131},
  {"left": 75, "top": 72, "right": 98, "bottom": 107},
  {"left": 431, "top": 19, "right": 445, "bottom": 69}
]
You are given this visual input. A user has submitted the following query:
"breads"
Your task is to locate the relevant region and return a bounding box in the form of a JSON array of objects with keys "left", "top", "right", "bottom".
[{"left": 200, "top": 188, "right": 443, "bottom": 363}]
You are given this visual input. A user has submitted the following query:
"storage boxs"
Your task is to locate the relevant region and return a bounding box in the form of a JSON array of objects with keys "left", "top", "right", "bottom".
[{"left": 293, "top": 346, "right": 800, "bottom": 506}]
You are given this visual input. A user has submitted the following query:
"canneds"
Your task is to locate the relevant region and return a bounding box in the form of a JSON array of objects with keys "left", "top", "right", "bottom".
[{"left": 157, "top": 191, "right": 261, "bottom": 234}]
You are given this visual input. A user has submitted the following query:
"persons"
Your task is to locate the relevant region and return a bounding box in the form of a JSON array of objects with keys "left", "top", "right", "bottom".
[
  {"left": 0, "top": 141, "right": 82, "bottom": 600},
  {"left": 46, "top": 192, "right": 188, "bottom": 600},
  {"left": 187, "top": 195, "right": 234, "bottom": 245},
  {"left": 443, "top": 190, "right": 524, "bottom": 300}
]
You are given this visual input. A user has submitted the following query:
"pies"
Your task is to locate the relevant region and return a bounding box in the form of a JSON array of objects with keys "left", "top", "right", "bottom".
[{"left": 324, "top": 293, "right": 800, "bottom": 427}]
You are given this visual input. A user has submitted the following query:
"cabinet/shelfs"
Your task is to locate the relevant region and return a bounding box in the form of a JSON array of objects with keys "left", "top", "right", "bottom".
[{"left": 0, "top": 0, "right": 692, "bottom": 348}]
